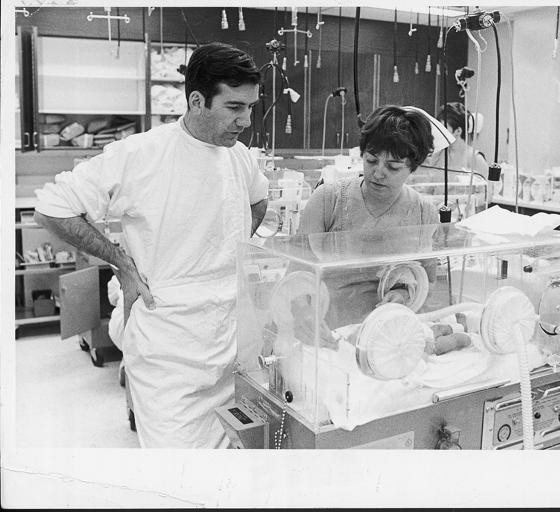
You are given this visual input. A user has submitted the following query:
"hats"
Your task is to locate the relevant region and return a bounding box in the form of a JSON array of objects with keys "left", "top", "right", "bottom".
[{"left": 398, "top": 105, "right": 456, "bottom": 153}]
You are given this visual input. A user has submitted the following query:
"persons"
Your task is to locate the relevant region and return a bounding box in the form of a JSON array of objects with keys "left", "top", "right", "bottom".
[
  {"left": 422, "top": 102, "right": 490, "bottom": 183},
  {"left": 33, "top": 43, "right": 268, "bottom": 448},
  {"left": 282, "top": 105, "right": 440, "bottom": 351}
]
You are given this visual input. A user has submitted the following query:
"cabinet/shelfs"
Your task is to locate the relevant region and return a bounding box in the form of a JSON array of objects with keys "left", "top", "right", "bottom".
[
  {"left": 31, "top": 24, "right": 151, "bottom": 152},
  {"left": 16, "top": 218, "right": 75, "bottom": 333},
  {"left": 14, "top": 27, "right": 32, "bottom": 151},
  {"left": 151, "top": 40, "right": 209, "bottom": 130}
]
